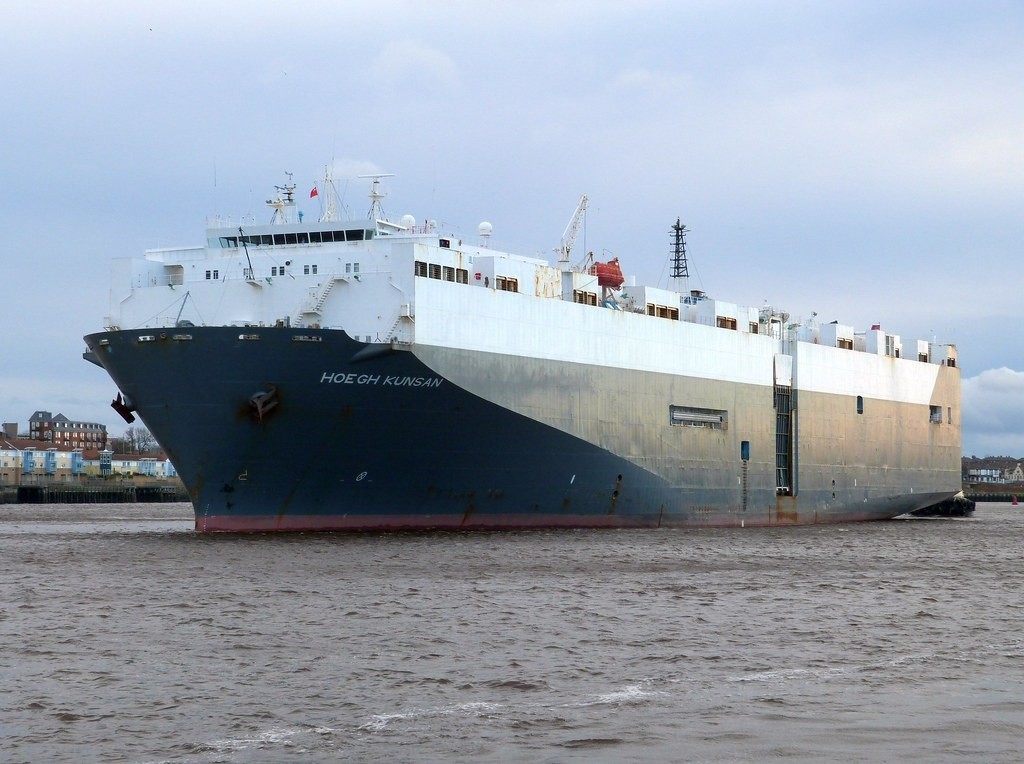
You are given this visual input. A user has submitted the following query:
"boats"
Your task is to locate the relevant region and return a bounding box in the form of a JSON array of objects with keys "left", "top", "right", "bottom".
[
  {"left": 911, "top": 498, "right": 975, "bottom": 518},
  {"left": 81, "top": 168, "right": 962, "bottom": 530}
]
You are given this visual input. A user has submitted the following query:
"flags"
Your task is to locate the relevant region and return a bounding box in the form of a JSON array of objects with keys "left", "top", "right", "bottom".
[{"left": 310, "top": 187, "right": 318, "bottom": 198}]
